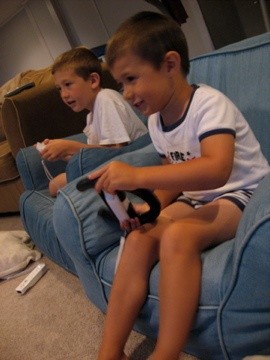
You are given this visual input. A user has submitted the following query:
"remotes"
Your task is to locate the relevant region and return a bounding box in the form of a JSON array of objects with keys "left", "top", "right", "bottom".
[{"left": 3, "top": 82, "right": 36, "bottom": 97}]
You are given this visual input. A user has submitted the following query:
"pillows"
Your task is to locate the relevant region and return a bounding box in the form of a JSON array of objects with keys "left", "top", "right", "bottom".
[{"left": 0, "top": 228, "right": 40, "bottom": 278}]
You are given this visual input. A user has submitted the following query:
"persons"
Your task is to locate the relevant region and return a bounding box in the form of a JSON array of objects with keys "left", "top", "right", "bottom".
[
  {"left": 34, "top": 46, "right": 149, "bottom": 198},
  {"left": 88, "top": 11, "right": 270, "bottom": 360}
]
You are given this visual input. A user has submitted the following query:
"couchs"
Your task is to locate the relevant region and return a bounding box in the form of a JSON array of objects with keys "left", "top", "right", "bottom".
[
  {"left": 14, "top": 109, "right": 153, "bottom": 278},
  {"left": 0, "top": 63, "right": 120, "bottom": 215},
  {"left": 49, "top": 33, "right": 270, "bottom": 360}
]
